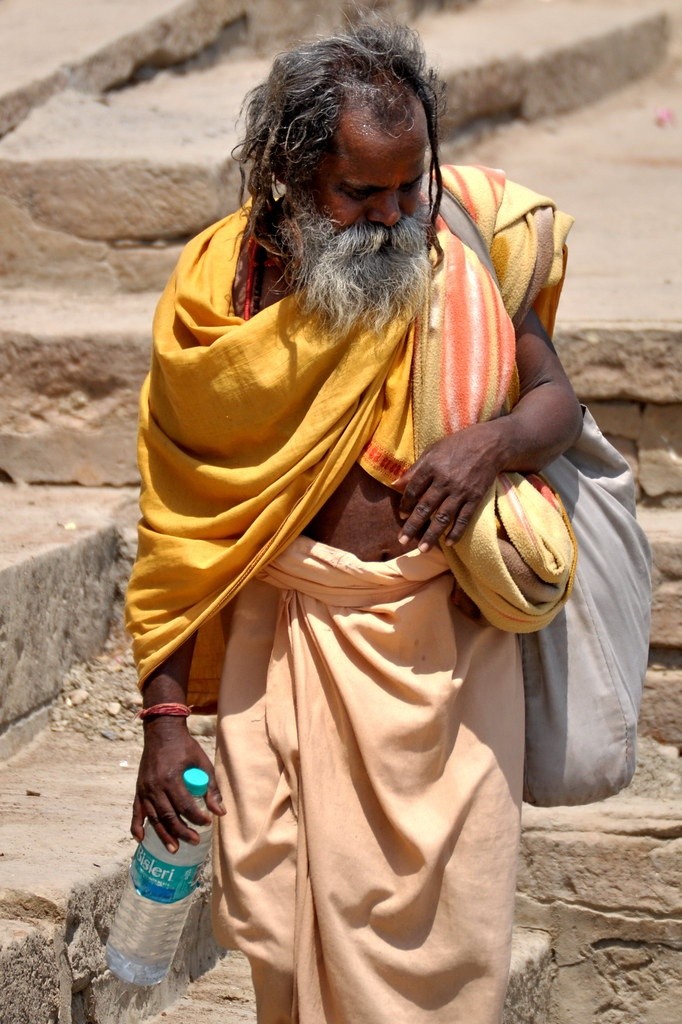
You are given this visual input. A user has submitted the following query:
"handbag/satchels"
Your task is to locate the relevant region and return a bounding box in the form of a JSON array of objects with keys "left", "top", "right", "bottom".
[{"left": 520, "top": 404, "right": 651, "bottom": 807}]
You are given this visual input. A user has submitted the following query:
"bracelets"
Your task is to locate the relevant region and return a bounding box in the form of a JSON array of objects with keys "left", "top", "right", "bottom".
[{"left": 134, "top": 702, "right": 194, "bottom": 720}]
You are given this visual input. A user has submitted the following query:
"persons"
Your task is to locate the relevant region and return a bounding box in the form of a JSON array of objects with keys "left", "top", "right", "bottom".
[{"left": 126, "top": 14, "right": 581, "bottom": 1024}]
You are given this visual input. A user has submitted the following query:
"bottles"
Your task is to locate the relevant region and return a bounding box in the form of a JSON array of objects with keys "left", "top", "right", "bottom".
[{"left": 106, "top": 768, "right": 214, "bottom": 987}]
